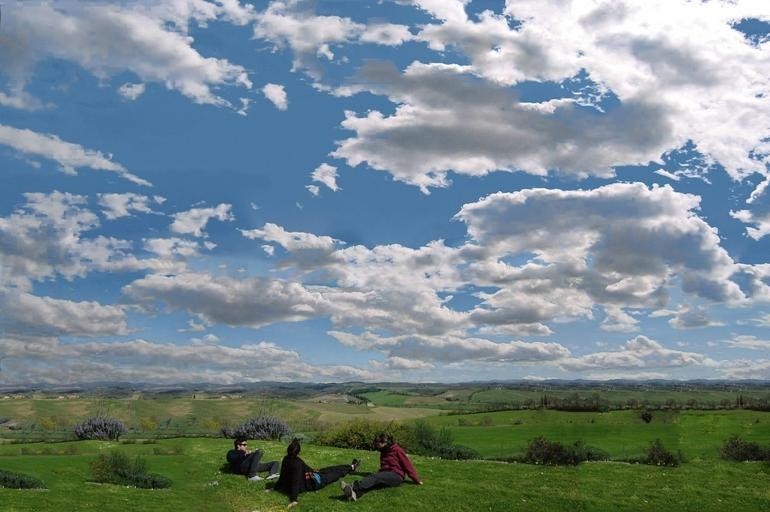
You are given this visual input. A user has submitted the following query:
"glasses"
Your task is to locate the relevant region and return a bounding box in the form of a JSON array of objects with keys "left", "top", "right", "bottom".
[{"left": 239, "top": 444, "right": 247, "bottom": 446}]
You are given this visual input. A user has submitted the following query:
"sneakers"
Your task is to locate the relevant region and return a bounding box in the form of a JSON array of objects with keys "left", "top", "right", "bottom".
[
  {"left": 248, "top": 476, "right": 264, "bottom": 481},
  {"left": 352, "top": 459, "right": 358, "bottom": 471},
  {"left": 266, "top": 473, "right": 280, "bottom": 480},
  {"left": 340, "top": 480, "right": 356, "bottom": 502}
]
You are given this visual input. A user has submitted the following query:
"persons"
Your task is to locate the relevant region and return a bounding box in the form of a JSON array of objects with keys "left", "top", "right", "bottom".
[
  {"left": 227, "top": 436, "right": 281, "bottom": 481},
  {"left": 341, "top": 429, "right": 423, "bottom": 500},
  {"left": 273, "top": 439, "right": 361, "bottom": 510}
]
